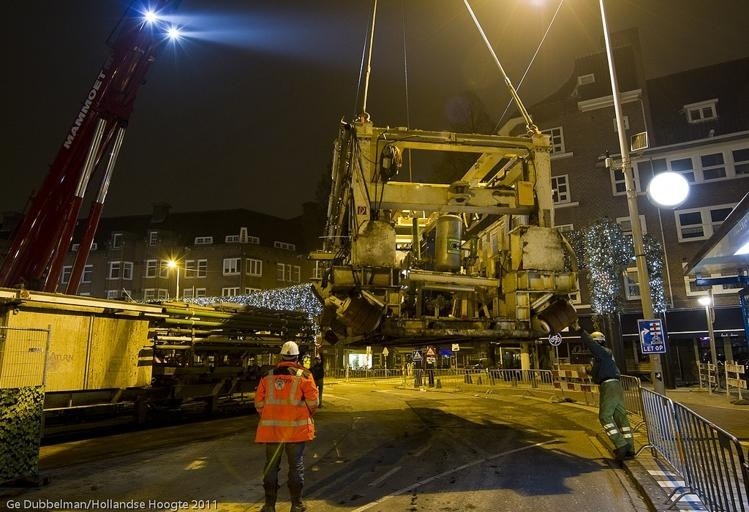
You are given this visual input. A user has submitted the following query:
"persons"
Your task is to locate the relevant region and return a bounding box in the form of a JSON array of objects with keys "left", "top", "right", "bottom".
[
  {"left": 571, "top": 318, "right": 635, "bottom": 463},
  {"left": 254, "top": 340, "right": 320, "bottom": 512},
  {"left": 308, "top": 356, "right": 324, "bottom": 408}
]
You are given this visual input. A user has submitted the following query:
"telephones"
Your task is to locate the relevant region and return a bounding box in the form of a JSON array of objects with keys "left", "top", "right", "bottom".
[{"left": 638, "top": 318, "right": 667, "bottom": 354}]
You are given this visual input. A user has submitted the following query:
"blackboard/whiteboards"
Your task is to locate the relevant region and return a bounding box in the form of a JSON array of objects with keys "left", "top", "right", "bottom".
[{"left": 308, "top": 121, "right": 577, "bottom": 346}]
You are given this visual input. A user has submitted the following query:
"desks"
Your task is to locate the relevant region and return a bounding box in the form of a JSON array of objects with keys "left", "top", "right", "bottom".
[{"left": 164, "top": 257, "right": 181, "bottom": 304}]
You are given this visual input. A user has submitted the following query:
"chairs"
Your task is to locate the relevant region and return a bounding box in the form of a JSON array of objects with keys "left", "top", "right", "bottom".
[
  {"left": 289, "top": 505, "right": 306, "bottom": 511},
  {"left": 260, "top": 505, "right": 276, "bottom": 511},
  {"left": 614, "top": 445, "right": 636, "bottom": 461}
]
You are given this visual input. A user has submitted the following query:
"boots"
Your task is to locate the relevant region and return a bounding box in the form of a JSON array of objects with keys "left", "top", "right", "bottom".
[{"left": 465, "top": 358, "right": 485, "bottom": 373}]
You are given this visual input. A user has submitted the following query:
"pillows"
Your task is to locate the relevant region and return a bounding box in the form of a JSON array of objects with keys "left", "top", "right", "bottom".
[
  {"left": 589, "top": 332, "right": 605, "bottom": 342},
  {"left": 280, "top": 340, "right": 299, "bottom": 356}
]
